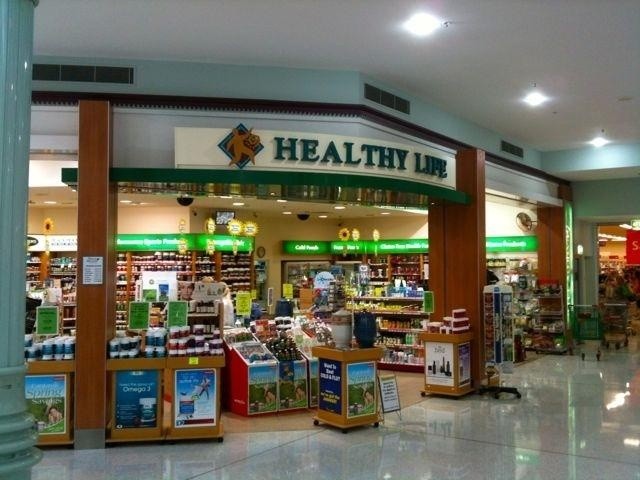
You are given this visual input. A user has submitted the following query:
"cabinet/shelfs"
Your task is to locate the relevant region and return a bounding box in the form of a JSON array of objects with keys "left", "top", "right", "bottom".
[
  {"left": 109, "top": 300, "right": 224, "bottom": 446},
  {"left": 27, "top": 234, "right": 254, "bottom": 336},
  {"left": 281, "top": 236, "right": 538, "bottom": 300},
  {"left": 530, "top": 285, "right": 567, "bottom": 353},
  {"left": 26, "top": 359, "right": 74, "bottom": 448},
  {"left": 312, "top": 342, "right": 382, "bottom": 432},
  {"left": 223, "top": 327, "right": 278, "bottom": 416},
  {"left": 286, "top": 330, "right": 319, "bottom": 408},
  {"left": 252, "top": 332, "right": 308, "bottom": 411},
  {"left": 354, "top": 295, "right": 424, "bottom": 372},
  {"left": 418, "top": 329, "right": 473, "bottom": 397}
]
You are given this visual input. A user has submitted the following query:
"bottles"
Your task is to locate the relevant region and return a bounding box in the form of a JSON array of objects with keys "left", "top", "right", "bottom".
[{"left": 266, "top": 330, "right": 302, "bottom": 362}]
